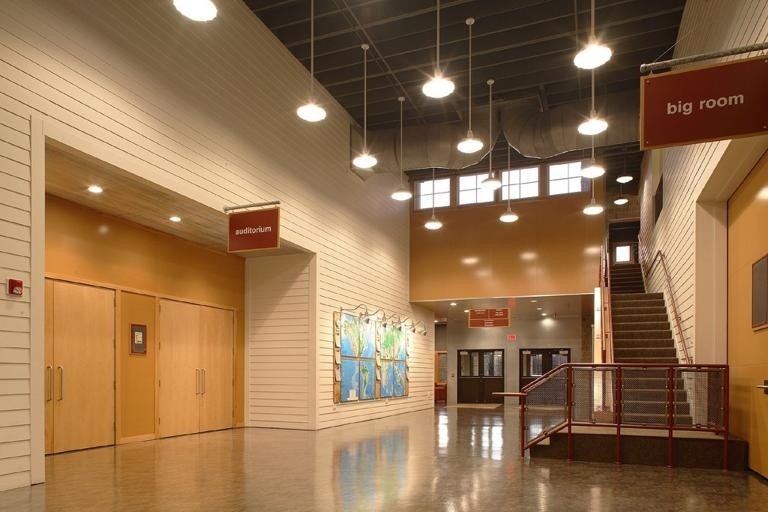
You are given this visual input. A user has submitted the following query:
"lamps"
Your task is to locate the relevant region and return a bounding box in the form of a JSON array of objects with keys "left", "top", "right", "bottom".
[
  {"left": 579, "top": 69, "right": 608, "bottom": 134},
  {"left": 422, "top": 155, "right": 445, "bottom": 232},
  {"left": 500, "top": 139, "right": 519, "bottom": 223},
  {"left": 340, "top": 303, "right": 427, "bottom": 336},
  {"left": 391, "top": 97, "right": 415, "bottom": 201},
  {"left": 422, "top": 2, "right": 453, "bottom": 100},
  {"left": 352, "top": 44, "right": 379, "bottom": 170},
  {"left": 580, "top": 137, "right": 606, "bottom": 177},
  {"left": 295, "top": 0, "right": 328, "bottom": 124},
  {"left": 582, "top": 179, "right": 605, "bottom": 216},
  {"left": 571, "top": 3, "right": 614, "bottom": 69},
  {"left": 457, "top": 16, "right": 484, "bottom": 153},
  {"left": 481, "top": 79, "right": 501, "bottom": 190}
]
